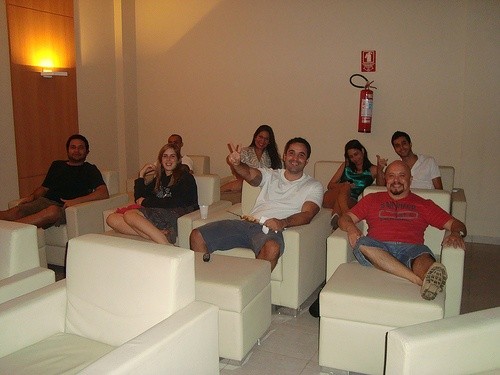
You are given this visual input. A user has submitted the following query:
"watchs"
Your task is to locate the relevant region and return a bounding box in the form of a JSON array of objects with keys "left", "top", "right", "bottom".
[{"left": 453, "top": 230, "right": 465, "bottom": 238}]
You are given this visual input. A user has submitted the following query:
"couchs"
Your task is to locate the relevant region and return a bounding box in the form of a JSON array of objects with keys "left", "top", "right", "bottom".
[
  {"left": 315, "top": 161, "right": 376, "bottom": 202},
  {"left": 439, "top": 166, "right": 467, "bottom": 223},
  {"left": 0, "top": 233, "right": 220, "bottom": 375},
  {"left": 103, "top": 175, "right": 232, "bottom": 249},
  {"left": 0, "top": 220, "right": 56, "bottom": 303},
  {"left": 193, "top": 180, "right": 333, "bottom": 317},
  {"left": 7, "top": 170, "right": 129, "bottom": 267},
  {"left": 327, "top": 185, "right": 465, "bottom": 319}
]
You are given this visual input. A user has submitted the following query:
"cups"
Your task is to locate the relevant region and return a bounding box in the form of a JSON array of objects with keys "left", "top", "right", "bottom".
[{"left": 199, "top": 204, "right": 209, "bottom": 219}]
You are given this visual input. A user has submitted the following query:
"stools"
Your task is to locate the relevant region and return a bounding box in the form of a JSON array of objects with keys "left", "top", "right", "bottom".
[
  {"left": 319, "top": 263, "right": 446, "bottom": 375},
  {"left": 195, "top": 252, "right": 271, "bottom": 361}
]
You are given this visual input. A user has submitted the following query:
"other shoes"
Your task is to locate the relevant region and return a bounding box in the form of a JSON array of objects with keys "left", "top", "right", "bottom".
[
  {"left": 330, "top": 213, "right": 341, "bottom": 232},
  {"left": 420, "top": 262, "right": 448, "bottom": 301}
]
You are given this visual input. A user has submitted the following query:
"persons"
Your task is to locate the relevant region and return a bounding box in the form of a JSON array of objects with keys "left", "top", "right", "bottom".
[
  {"left": 321, "top": 139, "right": 377, "bottom": 231},
  {"left": 0, "top": 134, "right": 109, "bottom": 225},
  {"left": 139, "top": 134, "right": 194, "bottom": 177},
  {"left": 189, "top": 136, "right": 324, "bottom": 273},
  {"left": 376, "top": 131, "right": 444, "bottom": 190},
  {"left": 221, "top": 124, "right": 283, "bottom": 194},
  {"left": 338, "top": 160, "right": 468, "bottom": 300},
  {"left": 107, "top": 144, "right": 201, "bottom": 248}
]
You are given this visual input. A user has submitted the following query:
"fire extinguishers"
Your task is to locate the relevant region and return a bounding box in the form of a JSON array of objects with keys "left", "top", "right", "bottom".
[{"left": 349, "top": 74, "right": 379, "bottom": 134}]
